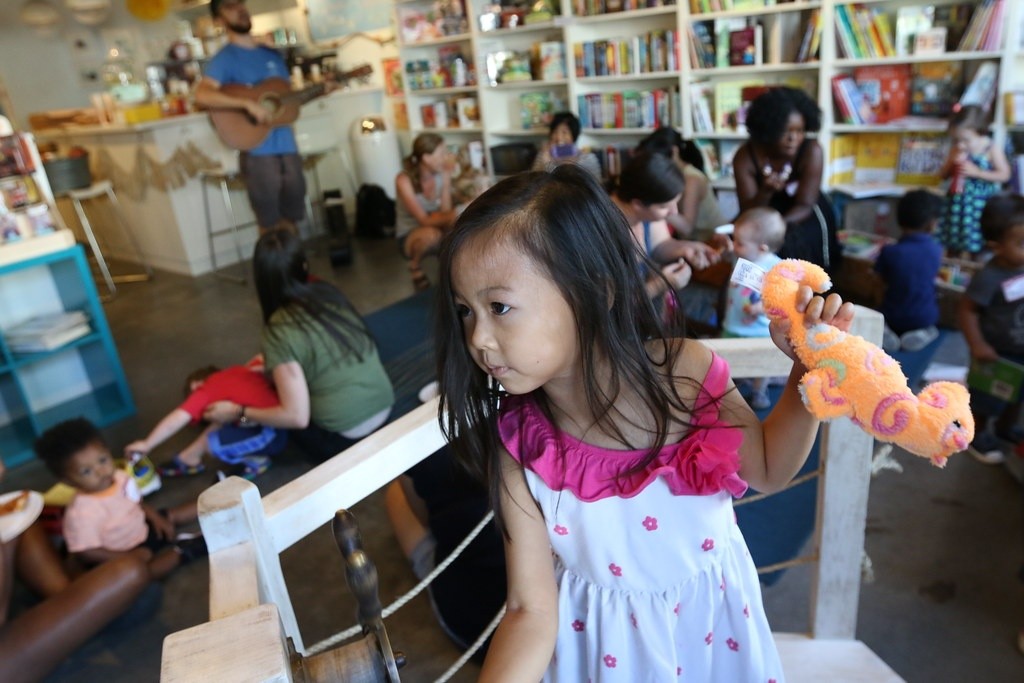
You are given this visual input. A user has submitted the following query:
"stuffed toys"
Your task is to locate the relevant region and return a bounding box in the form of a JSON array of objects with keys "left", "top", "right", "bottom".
[{"left": 760, "top": 257, "right": 977, "bottom": 470}]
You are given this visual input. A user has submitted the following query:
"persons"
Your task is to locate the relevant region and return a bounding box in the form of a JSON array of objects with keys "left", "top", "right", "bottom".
[
  {"left": 120, "top": 226, "right": 399, "bottom": 485},
  {"left": 420, "top": 163, "right": 856, "bottom": 683},
  {"left": 379, "top": 376, "right": 509, "bottom": 654},
  {"left": 594, "top": 125, "right": 796, "bottom": 407},
  {"left": 730, "top": 86, "right": 838, "bottom": 285},
  {"left": 391, "top": 131, "right": 483, "bottom": 290},
  {"left": 530, "top": 110, "right": 602, "bottom": 185},
  {"left": 0, "top": 416, "right": 208, "bottom": 683},
  {"left": 873, "top": 190, "right": 949, "bottom": 351},
  {"left": 936, "top": 100, "right": 1013, "bottom": 261},
  {"left": 952, "top": 191, "right": 1024, "bottom": 466},
  {"left": 194, "top": 1, "right": 349, "bottom": 240}
]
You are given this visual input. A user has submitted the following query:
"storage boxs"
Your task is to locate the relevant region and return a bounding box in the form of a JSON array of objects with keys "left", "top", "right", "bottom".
[
  {"left": 488, "top": 141, "right": 535, "bottom": 175},
  {"left": 113, "top": 102, "right": 161, "bottom": 127}
]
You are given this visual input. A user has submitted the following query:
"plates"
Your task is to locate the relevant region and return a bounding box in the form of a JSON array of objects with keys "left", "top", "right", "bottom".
[{"left": 0, "top": 490, "right": 44, "bottom": 544}]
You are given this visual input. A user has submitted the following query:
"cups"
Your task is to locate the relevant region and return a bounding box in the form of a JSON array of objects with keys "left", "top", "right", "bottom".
[{"left": 93, "top": 28, "right": 324, "bottom": 126}]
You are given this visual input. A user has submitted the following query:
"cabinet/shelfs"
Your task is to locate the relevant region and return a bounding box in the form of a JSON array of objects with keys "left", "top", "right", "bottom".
[
  {"left": 391, "top": 0, "right": 1024, "bottom": 200},
  {"left": 0, "top": 241, "right": 140, "bottom": 471}
]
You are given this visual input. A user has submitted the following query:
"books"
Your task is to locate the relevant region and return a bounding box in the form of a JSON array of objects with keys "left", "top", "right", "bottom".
[
  {"left": 688, "top": 0, "right": 826, "bottom": 68},
  {"left": 833, "top": 61, "right": 999, "bottom": 124},
  {"left": 518, "top": 40, "right": 568, "bottom": 129},
  {"left": 828, "top": 133, "right": 951, "bottom": 191},
  {"left": 834, "top": 0, "right": 1007, "bottom": 58},
  {"left": 571, "top": 0, "right": 681, "bottom": 77},
  {"left": 686, "top": 75, "right": 819, "bottom": 180},
  {"left": 398, "top": 0, "right": 484, "bottom": 169},
  {"left": 577, "top": 83, "right": 682, "bottom": 179}
]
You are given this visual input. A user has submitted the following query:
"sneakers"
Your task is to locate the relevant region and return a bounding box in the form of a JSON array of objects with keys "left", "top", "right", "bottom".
[{"left": 176, "top": 531, "right": 208, "bottom": 564}]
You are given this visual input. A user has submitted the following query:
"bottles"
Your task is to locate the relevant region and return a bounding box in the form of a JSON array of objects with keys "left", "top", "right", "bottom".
[
  {"left": 950, "top": 154, "right": 967, "bottom": 194},
  {"left": 874, "top": 203, "right": 890, "bottom": 236}
]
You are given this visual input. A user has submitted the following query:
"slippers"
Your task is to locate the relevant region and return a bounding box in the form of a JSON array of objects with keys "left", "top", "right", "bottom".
[
  {"left": 158, "top": 453, "right": 204, "bottom": 476},
  {"left": 239, "top": 456, "right": 272, "bottom": 480}
]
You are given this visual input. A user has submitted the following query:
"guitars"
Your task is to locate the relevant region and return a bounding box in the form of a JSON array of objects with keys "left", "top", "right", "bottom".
[{"left": 206, "top": 62, "right": 375, "bottom": 151}]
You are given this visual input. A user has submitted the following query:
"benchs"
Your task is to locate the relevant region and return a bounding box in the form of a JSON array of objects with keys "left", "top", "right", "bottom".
[{"left": 157, "top": 303, "right": 912, "bottom": 683}]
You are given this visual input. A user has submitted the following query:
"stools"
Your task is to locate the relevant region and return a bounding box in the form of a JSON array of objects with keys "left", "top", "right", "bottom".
[
  {"left": 198, "top": 162, "right": 261, "bottom": 283},
  {"left": 52, "top": 180, "right": 152, "bottom": 303},
  {"left": 295, "top": 146, "right": 360, "bottom": 258}
]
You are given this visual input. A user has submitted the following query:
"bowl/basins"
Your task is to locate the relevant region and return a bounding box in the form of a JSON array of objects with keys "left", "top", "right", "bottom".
[{"left": 42, "top": 154, "right": 91, "bottom": 192}]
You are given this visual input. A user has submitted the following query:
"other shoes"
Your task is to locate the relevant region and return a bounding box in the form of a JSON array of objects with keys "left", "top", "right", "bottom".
[
  {"left": 999, "top": 433, "right": 1022, "bottom": 447},
  {"left": 901, "top": 325, "right": 940, "bottom": 351},
  {"left": 883, "top": 324, "right": 901, "bottom": 353},
  {"left": 967, "top": 430, "right": 1005, "bottom": 463}
]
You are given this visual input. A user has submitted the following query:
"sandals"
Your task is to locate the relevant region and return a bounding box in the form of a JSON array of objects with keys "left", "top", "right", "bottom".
[{"left": 409, "top": 261, "right": 430, "bottom": 289}]
